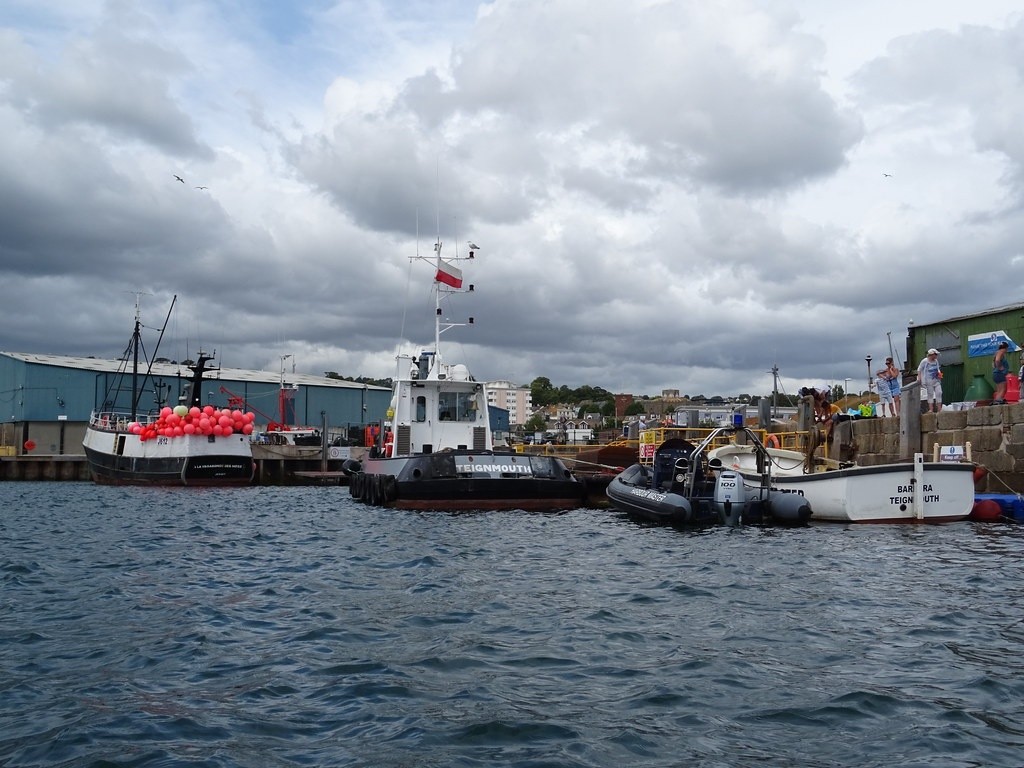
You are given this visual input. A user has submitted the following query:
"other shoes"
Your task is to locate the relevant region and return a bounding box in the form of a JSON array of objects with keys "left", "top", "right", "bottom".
[
  {"left": 927, "top": 411, "right": 942, "bottom": 413},
  {"left": 882, "top": 412, "right": 900, "bottom": 417},
  {"left": 995, "top": 399, "right": 1006, "bottom": 404}
]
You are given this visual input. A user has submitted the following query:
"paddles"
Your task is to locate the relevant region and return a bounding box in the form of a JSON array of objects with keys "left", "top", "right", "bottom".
[{"left": 558, "top": 456, "right": 626, "bottom": 472}]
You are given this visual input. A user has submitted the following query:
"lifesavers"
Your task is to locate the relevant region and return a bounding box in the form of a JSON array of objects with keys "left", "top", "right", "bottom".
[
  {"left": 24, "top": 440, "right": 36, "bottom": 450},
  {"left": 387, "top": 432, "right": 394, "bottom": 456},
  {"left": 763, "top": 434, "right": 779, "bottom": 450}
]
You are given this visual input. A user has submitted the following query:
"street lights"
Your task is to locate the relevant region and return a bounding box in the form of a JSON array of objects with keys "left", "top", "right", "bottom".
[
  {"left": 845, "top": 378, "right": 852, "bottom": 411},
  {"left": 865, "top": 355, "right": 871, "bottom": 404}
]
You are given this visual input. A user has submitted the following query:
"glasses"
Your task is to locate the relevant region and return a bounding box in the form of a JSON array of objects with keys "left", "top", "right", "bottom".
[
  {"left": 885, "top": 362, "right": 891, "bottom": 365},
  {"left": 935, "top": 354, "right": 938, "bottom": 355}
]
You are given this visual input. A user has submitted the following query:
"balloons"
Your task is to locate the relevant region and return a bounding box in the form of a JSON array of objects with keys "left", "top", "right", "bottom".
[{"left": 129, "top": 404, "right": 256, "bottom": 441}]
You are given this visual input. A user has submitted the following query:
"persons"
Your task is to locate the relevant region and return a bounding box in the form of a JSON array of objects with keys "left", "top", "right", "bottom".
[
  {"left": 798, "top": 387, "right": 810, "bottom": 399},
  {"left": 884, "top": 358, "right": 900, "bottom": 420},
  {"left": 818, "top": 401, "right": 844, "bottom": 423},
  {"left": 809, "top": 387, "right": 828, "bottom": 422},
  {"left": 917, "top": 348, "right": 943, "bottom": 413},
  {"left": 991, "top": 342, "right": 1010, "bottom": 404},
  {"left": 871, "top": 371, "right": 896, "bottom": 418}
]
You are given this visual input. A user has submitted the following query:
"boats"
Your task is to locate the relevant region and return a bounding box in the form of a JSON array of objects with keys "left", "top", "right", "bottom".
[
  {"left": 973, "top": 490, "right": 1024, "bottom": 524},
  {"left": 602, "top": 437, "right": 814, "bottom": 531},
  {"left": 248, "top": 350, "right": 372, "bottom": 487},
  {"left": 705, "top": 436, "right": 976, "bottom": 524},
  {"left": 347, "top": 152, "right": 604, "bottom": 512}
]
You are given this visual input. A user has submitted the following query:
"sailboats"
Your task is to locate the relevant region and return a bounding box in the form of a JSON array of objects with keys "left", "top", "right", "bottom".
[{"left": 82, "top": 288, "right": 255, "bottom": 491}]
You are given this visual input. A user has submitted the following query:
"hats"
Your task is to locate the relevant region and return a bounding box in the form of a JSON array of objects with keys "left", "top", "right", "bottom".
[{"left": 928, "top": 348, "right": 941, "bottom": 354}]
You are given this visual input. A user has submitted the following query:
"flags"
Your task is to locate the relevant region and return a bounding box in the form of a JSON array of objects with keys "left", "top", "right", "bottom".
[{"left": 434, "top": 258, "right": 463, "bottom": 288}]
